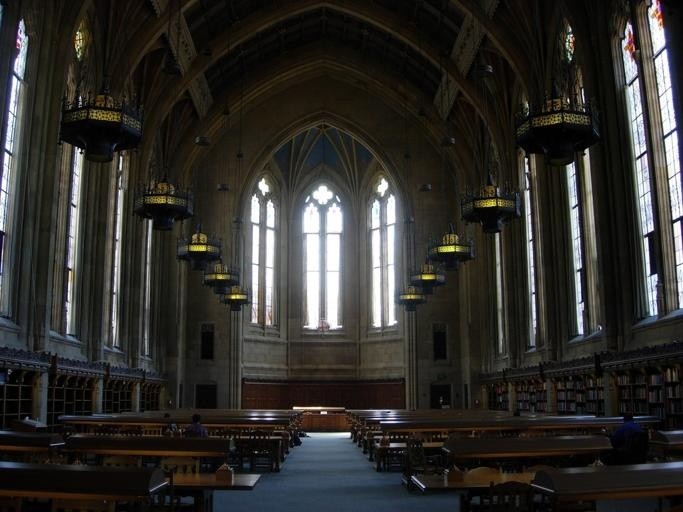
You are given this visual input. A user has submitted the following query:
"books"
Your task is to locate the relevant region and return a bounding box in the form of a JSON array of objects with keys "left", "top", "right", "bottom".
[
  {"left": 555, "top": 375, "right": 604, "bottom": 415},
  {"left": 492, "top": 383, "right": 509, "bottom": 410},
  {"left": 516, "top": 382, "right": 546, "bottom": 411},
  {"left": 615, "top": 368, "right": 683, "bottom": 429}
]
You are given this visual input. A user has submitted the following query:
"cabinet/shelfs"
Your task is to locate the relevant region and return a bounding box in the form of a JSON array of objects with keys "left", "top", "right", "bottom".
[
  {"left": 484, "top": 342, "right": 682, "bottom": 422},
  {"left": 0, "top": 348, "right": 168, "bottom": 424}
]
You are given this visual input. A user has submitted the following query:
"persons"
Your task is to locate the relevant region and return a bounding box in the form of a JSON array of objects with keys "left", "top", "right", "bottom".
[
  {"left": 164, "top": 412, "right": 177, "bottom": 432},
  {"left": 183, "top": 414, "right": 210, "bottom": 438},
  {"left": 609, "top": 410, "right": 649, "bottom": 466}
]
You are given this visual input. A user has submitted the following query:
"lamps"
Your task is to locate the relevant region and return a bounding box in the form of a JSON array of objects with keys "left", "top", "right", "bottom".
[
  {"left": 57, "top": 1, "right": 148, "bottom": 164},
  {"left": 133, "top": 4, "right": 246, "bottom": 312},
  {"left": 511, "top": 2, "right": 604, "bottom": 167},
  {"left": 397, "top": 4, "right": 521, "bottom": 313}
]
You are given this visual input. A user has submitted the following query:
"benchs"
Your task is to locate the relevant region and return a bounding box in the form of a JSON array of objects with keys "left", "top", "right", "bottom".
[
  {"left": 1, "top": 412, "right": 302, "bottom": 509},
  {"left": 345, "top": 408, "right": 680, "bottom": 511}
]
